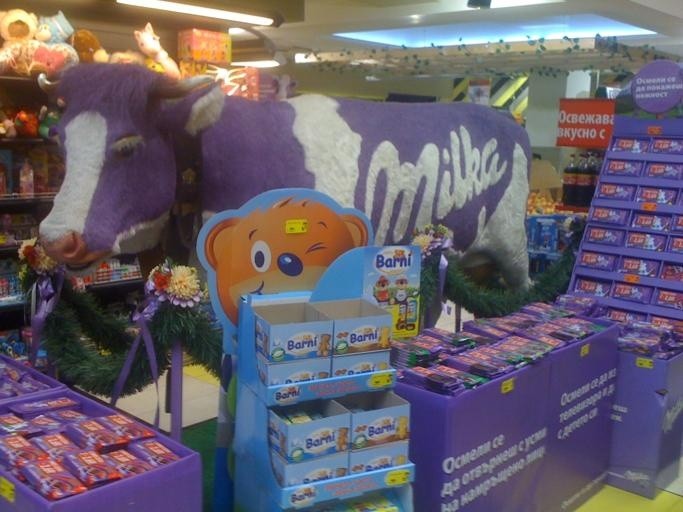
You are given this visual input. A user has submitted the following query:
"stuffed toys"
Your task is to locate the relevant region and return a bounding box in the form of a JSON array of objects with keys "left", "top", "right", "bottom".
[{"left": 0, "top": 10, "right": 181, "bottom": 146}]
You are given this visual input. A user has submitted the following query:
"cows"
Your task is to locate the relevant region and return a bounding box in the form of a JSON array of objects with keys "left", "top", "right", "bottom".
[{"left": 30, "top": 55, "right": 538, "bottom": 453}]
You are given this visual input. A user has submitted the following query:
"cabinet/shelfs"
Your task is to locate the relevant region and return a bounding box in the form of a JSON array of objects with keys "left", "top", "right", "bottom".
[
  {"left": 1, "top": 59, "right": 149, "bottom": 326},
  {"left": 230, "top": 292, "right": 416, "bottom": 512},
  {"left": 390, "top": 340, "right": 552, "bottom": 512},
  {"left": 0, "top": 355, "right": 66, "bottom": 401},
  {"left": 524, "top": 119, "right": 682, "bottom": 500},
  {"left": 471, "top": 308, "right": 621, "bottom": 512}
]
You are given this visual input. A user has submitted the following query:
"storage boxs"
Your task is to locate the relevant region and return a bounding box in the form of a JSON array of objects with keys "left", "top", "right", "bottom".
[{"left": 177, "top": 28, "right": 233, "bottom": 64}]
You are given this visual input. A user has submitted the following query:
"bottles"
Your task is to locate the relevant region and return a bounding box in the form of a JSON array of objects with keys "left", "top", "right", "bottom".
[{"left": 561, "top": 151, "right": 607, "bottom": 205}]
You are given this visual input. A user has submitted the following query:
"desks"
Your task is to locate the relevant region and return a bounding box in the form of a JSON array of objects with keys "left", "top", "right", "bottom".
[{"left": 0, "top": 388, "right": 203, "bottom": 510}]
